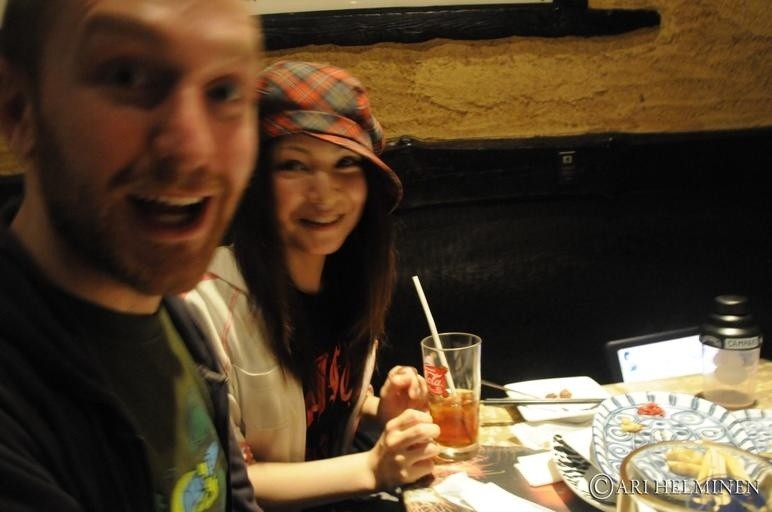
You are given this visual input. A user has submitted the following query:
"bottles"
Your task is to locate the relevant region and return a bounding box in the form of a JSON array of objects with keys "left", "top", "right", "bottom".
[{"left": 700, "top": 296, "right": 762, "bottom": 409}]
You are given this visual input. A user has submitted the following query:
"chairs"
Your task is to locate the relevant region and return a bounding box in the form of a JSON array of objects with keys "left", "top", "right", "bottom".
[{"left": 605, "top": 325, "right": 718, "bottom": 384}]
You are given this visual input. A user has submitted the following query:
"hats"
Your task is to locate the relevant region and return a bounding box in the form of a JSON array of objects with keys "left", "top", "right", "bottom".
[{"left": 256, "top": 60, "right": 404, "bottom": 214}]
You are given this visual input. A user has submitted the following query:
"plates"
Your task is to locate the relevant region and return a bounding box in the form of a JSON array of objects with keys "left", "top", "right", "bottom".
[{"left": 505, "top": 373, "right": 772, "bottom": 511}]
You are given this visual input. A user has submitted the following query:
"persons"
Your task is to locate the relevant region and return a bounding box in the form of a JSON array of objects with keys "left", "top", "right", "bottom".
[
  {"left": 0, "top": 0, "right": 265, "bottom": 512},
  {"left": 186, "top": 61, "right": 442, "bottom": 511}
]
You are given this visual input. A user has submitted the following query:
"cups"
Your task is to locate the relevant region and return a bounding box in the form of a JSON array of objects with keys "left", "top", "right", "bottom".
[{"left": 421, "top": 333, "right": 485, "bottom": 459}]
